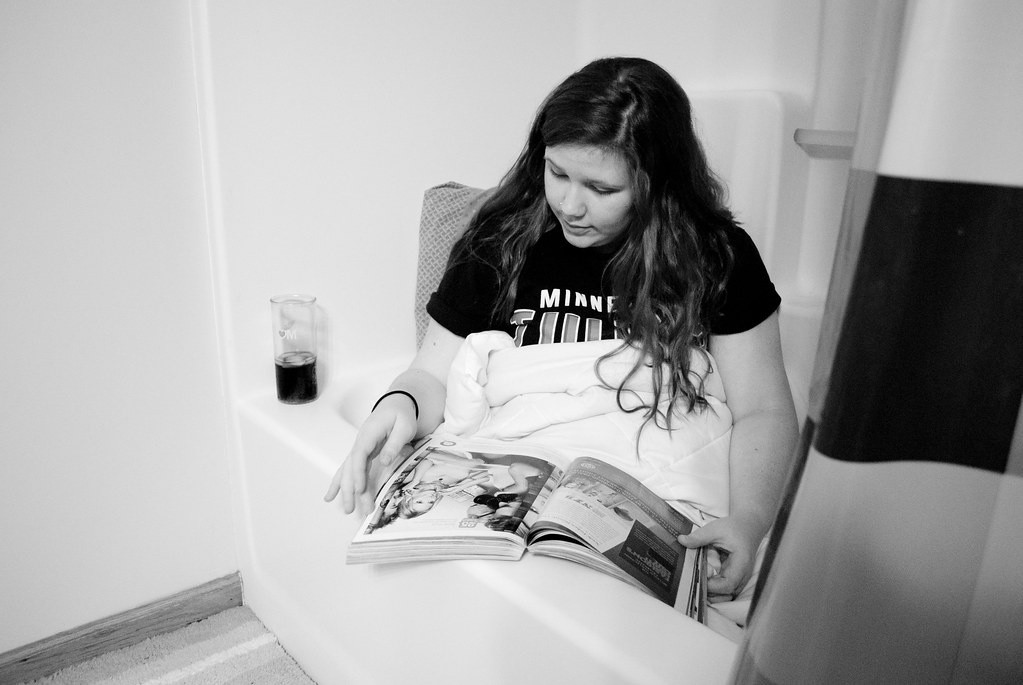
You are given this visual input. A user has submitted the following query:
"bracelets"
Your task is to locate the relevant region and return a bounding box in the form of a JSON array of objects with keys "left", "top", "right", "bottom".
[{"left": 372, "top": 390, "right": 419, "bottom": 418}]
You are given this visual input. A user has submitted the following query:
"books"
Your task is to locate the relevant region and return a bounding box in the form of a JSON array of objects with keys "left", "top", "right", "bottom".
[{"left": 346, "top": 435, "right": 709, "bottom": 625}]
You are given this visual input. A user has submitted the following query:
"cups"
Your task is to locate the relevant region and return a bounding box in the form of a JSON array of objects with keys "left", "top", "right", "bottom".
[{"left": 271, "top": 294, "right": 317, "bottom": 403}]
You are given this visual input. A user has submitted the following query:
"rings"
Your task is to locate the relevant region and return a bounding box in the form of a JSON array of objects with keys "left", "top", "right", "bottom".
[{"left": 732, "top": 592, "right": 737, "bottom": 601}]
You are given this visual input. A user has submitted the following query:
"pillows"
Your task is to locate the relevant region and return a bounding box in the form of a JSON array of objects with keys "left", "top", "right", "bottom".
[{"left": 414, "top": 179, "right": 505, "bottom": 355}]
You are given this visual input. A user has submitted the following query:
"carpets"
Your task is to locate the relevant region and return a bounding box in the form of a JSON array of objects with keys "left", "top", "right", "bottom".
[{"left": 18, "top": 605, "right": 318, "bottom": 685}]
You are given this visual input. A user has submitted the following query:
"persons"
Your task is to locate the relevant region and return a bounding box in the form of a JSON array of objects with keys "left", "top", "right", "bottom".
[
  {"left": 323, "top": 56, "right": 801, "bottom": 604},
  {"left": 372, "top": 448, "right": 542, "bottom": 529}
]
